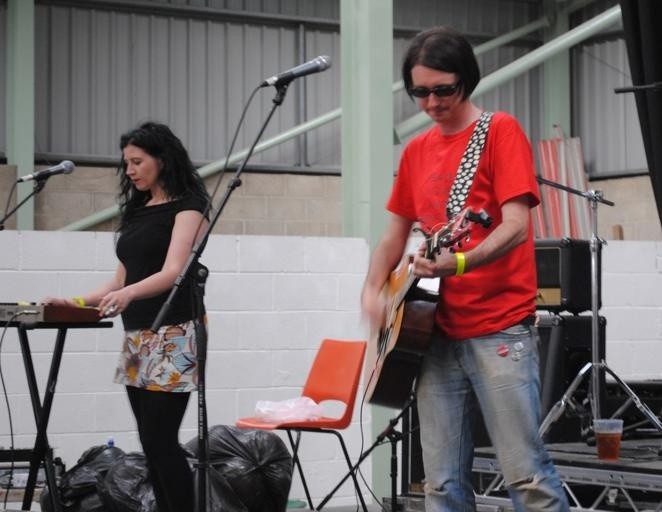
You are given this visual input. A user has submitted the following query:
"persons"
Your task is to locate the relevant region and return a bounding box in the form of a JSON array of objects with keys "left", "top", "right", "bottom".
[
  {"left": 45, "top": 122, "right": 213, "bottom": 510},
  {"left": 359, "top": 23, "right": 573, "bottom": 510}
]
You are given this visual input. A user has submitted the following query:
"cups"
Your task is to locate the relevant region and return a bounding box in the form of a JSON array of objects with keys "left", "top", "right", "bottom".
[{"left": 592, "top": 418, "right": 625, "bottom": 462}]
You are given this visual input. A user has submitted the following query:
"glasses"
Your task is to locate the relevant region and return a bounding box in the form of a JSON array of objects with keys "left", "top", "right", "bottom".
[{"left": 408, "top": 81, "right": 462, "bottom": 98}]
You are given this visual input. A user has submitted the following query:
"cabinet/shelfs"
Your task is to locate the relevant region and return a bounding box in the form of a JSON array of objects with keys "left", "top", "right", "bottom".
[{"left": 1, "top": 324, "right": 113, "bottom": 511}]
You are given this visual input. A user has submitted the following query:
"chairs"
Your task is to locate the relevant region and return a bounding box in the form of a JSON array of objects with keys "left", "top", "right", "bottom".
[{"left": 235, "top": 338, "right": 369, "bottom": 511}]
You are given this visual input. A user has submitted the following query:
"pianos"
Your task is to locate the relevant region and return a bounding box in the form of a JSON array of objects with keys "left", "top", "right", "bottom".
[{"left": 0, "top": 301, "right": 103, "bottom": 323}]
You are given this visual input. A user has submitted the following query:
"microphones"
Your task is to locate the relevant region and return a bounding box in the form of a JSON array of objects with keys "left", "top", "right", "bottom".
[
  {"left": 260, "top": 54, "right": 333, "bottom": 88},
  {"left": 17, "top": 159, "right": 75, "bottom": 184}
]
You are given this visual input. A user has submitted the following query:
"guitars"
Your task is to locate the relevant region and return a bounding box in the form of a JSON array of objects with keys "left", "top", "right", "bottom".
[{"left": 363, "top": 207, "right": 493, "bottom": 408}]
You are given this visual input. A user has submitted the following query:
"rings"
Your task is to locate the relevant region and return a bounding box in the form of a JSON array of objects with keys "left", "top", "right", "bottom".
[{"left": 108, "top": 304, "right": 118, "bottom": 312}]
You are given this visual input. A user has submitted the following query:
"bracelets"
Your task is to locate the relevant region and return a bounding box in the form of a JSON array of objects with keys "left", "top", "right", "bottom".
[
  {"left": 451, "top": 248, "right": 468, "bottom": 274},
  {"left": 71, "top": 293, "right": 86, "bottom": 307}
]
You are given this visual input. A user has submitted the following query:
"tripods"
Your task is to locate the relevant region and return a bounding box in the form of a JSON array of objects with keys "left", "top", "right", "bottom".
[{"left": 481, "top": 189, "right": 662, "bottom": 498}]
